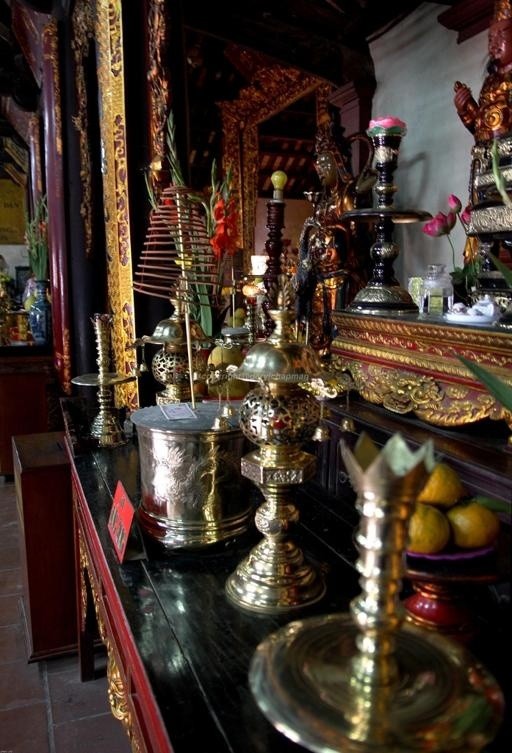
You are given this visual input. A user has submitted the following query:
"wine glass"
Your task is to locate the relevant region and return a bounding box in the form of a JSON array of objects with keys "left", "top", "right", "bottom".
[{"left": 401, "top": 546, "right": 503, "bottom": 635}]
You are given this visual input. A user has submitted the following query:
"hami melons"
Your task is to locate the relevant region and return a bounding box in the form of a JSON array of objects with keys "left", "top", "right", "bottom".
[{"left": 207, "top": 346, "right": 250, "bottom": 398}]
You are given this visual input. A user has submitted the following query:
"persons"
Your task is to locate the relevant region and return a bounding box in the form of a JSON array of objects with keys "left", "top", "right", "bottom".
[
  {"left": 302, "top": 133, "right": 359, "bottom": 364},
  {"left": 449, "top": 0, "right": 511, "bottom": 289}
]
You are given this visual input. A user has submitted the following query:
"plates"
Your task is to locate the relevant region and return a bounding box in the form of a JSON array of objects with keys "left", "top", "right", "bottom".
[{"left": 444, "top": 313, "right": 500, "bottom": 325}]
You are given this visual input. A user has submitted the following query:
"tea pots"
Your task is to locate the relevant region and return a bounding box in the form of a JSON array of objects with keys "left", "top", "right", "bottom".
[{"left": 472, "top": 294, "right": 498, "bottom": 318}]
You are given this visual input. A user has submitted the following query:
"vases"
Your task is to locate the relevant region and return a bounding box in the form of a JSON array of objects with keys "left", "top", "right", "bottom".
[
  {"left": 192, "top": 348, "right": 213, "bottom": 397},
  {"left": 21, "top": 278, "right": 54, "bottom": 345}
]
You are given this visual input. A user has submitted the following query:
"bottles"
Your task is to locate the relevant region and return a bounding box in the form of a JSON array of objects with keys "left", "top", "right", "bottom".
[{"left": 407, "top": 262, "right": 455, "bottom": 322}]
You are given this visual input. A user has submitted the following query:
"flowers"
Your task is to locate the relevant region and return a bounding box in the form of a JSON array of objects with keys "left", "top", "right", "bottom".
[
  {"left": 422, "top": 191, "right": 486, "bottom": 291},
  {"left": 143, "top": 109, "right": 241, "bottom": 348},
  {"left": 24, "top": 193, "right": 49, "bottom": 280}
]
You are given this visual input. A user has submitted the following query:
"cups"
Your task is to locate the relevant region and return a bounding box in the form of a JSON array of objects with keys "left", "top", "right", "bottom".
[{"left": 451, "top": 302, "right": 467, "bottom": 316}]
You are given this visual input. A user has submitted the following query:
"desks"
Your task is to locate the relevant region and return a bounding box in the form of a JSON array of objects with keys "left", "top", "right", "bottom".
[{"left": 60, "top": 392, "right": 511, "bottom": 752}]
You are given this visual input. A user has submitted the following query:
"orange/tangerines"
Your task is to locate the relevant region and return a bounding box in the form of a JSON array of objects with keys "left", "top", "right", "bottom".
[
  {"left": 227, "top": 308, "right": 247, "bottom": 328},
  {"left": 406, "top": 463, "right": 499, "bottom": 555}
]
